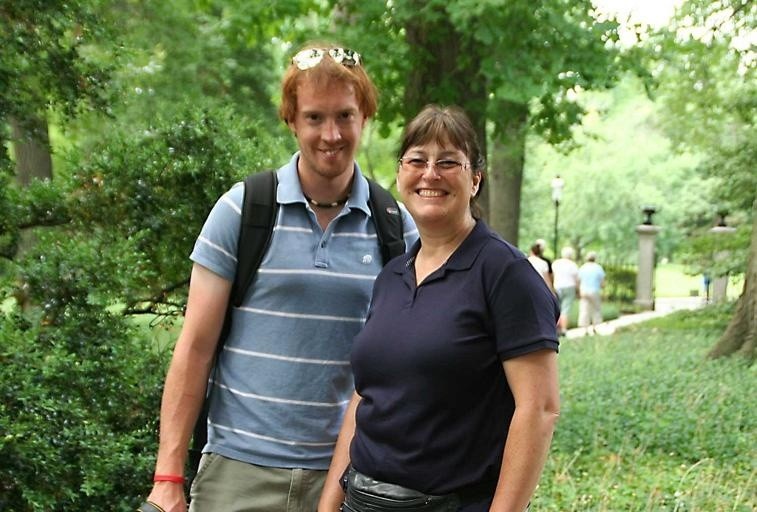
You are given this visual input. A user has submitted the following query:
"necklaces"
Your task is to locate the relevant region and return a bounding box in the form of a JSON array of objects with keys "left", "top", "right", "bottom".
[{"left": 302, "top": 191, "right": 351, "bottom": 208}]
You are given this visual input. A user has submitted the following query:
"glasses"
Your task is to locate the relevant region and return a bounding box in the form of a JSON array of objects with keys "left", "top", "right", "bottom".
[
  {"left": 399, "top": 157, "right": 471, "bottom": 173},
  {"left": 292, "top": 48, "right": 362, "bottom": 71}
]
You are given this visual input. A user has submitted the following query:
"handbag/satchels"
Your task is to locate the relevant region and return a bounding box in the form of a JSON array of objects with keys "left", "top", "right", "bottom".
[{"left": 339, "top": 461, "right": 461, "bottom": 512}]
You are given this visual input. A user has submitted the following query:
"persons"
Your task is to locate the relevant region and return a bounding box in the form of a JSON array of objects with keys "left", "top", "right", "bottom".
[
  {"left": 577, "top": 250, "right": 607, "bottom": 336},
  {"left": 526, "top": 244, "right": 557, "bottom": 298},
  {"left": 536, "top": 239, "right": 554, "bottom": 286},
  {"left": 550, "top": 247, "right": 581, "bottom": 338},
  {"left": 140, "top": 43, "right": 420, "bottom": 512},
  {"left": 314, "top": 103, "right": 562, "bottom": 510}
]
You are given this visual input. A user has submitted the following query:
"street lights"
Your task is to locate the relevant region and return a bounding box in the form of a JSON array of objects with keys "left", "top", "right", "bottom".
[{"left": 553, "top": 175, "right": 563, "bottom": 260}]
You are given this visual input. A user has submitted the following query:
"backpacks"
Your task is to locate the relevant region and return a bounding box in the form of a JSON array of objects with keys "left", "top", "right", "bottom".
[{"left": 187, "top": 169, "right": 406, "bottom": 469}]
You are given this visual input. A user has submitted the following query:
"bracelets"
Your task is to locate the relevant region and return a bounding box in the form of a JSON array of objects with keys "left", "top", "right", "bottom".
[{"left": 152, "top": 474, "right": 186, "bottom": 483}]
